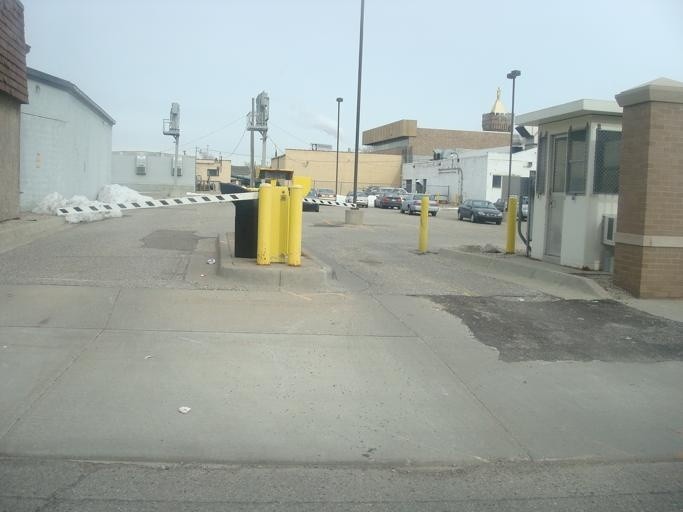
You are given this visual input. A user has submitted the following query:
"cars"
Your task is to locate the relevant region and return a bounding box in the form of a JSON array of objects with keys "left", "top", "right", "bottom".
[{"left": 305, "top": 183, "right": 530, "bottom": 227}]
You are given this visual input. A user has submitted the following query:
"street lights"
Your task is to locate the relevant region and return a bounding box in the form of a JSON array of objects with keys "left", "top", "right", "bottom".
[
  {"left": 335, "top": 97, "right": 343, "bottom": 196},
  {"left": 505, "top": 69, "right": 522, "bottom": 212}
]
